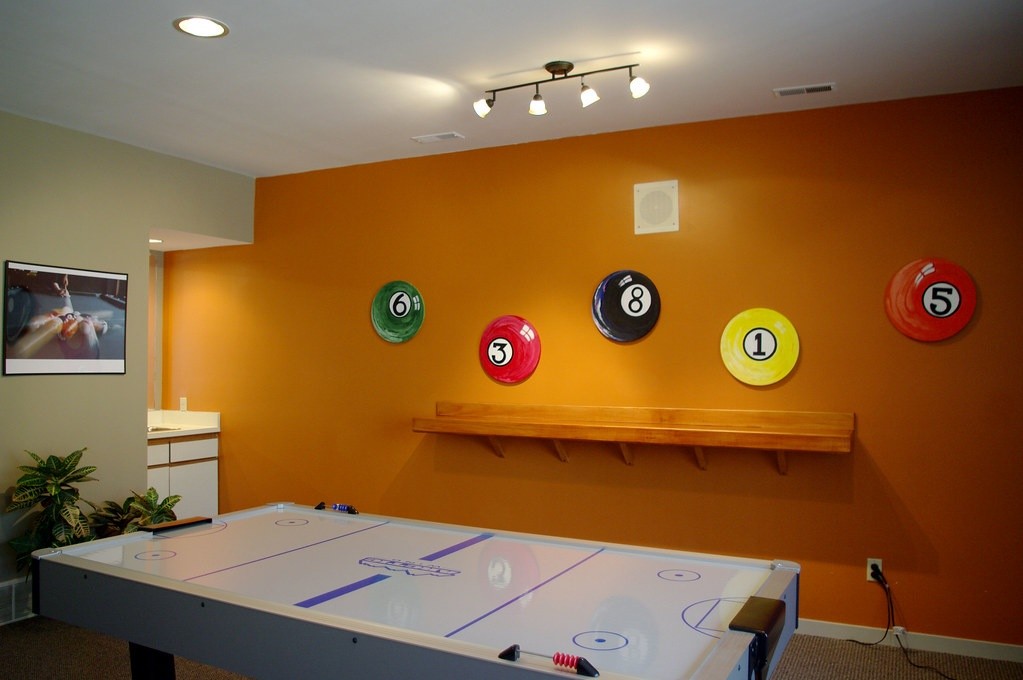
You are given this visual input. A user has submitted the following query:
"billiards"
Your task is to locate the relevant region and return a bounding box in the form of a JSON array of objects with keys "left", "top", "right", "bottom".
[{"left": 29, "top": 305, "right": 113, "bottom": 341}]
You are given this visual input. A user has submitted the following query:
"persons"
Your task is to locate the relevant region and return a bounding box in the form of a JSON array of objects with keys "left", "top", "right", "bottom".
[{"left": 36, "top": 271, "right": 69, "bottom": 297}]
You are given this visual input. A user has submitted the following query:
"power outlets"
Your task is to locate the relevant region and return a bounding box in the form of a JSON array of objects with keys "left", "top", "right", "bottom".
[{"left": 867, "top": 558, "right": 883, "bottom": 582}]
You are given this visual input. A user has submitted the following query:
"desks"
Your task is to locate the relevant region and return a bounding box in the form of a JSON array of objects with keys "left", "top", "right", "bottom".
[
  {"left": 413, "top": 401, "right": 855, "bottom": 474},
  {"left": 30, "top": 502, "right": 801, "bottom": 680}
]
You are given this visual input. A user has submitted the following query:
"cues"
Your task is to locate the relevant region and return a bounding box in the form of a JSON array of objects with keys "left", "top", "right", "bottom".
[
  {"left": 11, "top": 318, "right": 64, "bottom": 358},
  {"left": 65, "top": 285, "right": 74, "bottom": 311}
]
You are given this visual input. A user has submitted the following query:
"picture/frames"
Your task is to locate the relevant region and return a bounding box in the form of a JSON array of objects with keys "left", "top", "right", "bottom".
[{"left": 3, "top": 260, "right": 129, "bottom": 374}]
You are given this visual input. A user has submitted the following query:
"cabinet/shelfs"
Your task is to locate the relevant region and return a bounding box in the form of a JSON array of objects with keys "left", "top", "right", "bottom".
[{"left": 147, "top": 434, "right": 218, "bottom": 523}]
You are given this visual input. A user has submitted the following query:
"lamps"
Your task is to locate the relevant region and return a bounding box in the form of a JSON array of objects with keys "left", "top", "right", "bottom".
[
  {"left": 529, "top": 84, "right": 547, "bottom": 116},
  {"left": 581, "top": 75, "right": 600, "bottom": 108},
  {"left": 472, "top": 92, "right": 496, "bottom": 118},
  {"left": 629, "top": 66, "right": 650, "bottom": 98}
]
